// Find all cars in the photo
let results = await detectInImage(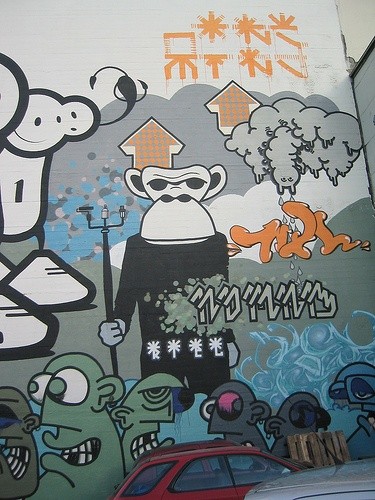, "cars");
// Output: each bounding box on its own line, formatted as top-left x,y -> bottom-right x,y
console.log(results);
242,458 -> 375,500
106,440 -> 309,500
